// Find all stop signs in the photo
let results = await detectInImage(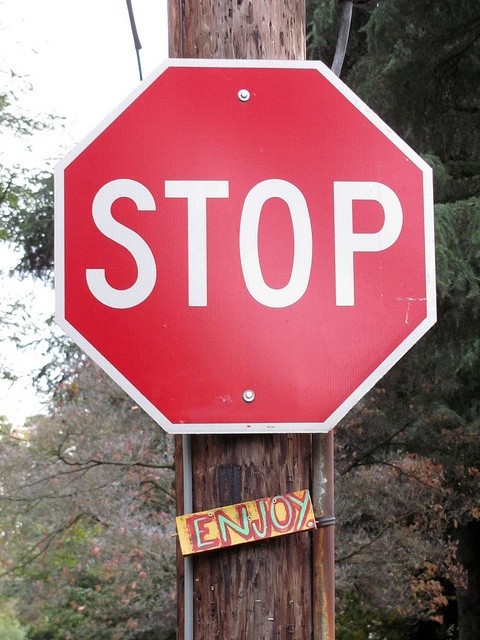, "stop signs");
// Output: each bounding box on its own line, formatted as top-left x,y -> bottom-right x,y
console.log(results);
54,59 -> 437,435
